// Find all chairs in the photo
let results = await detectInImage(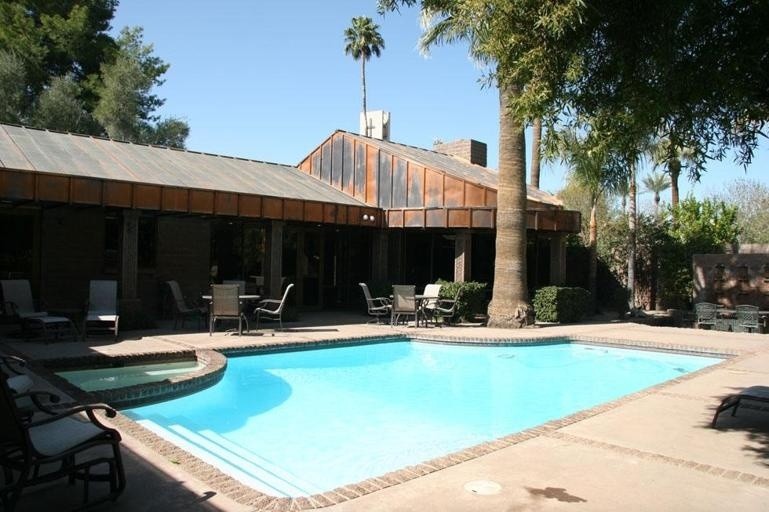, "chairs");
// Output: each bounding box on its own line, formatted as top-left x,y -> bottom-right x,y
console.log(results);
677,301 -> 769,334
0,278 -> 119,344
166,279 -> 295,335
712,386 -> 769,427
359,281 -> 463,328
0,353 -> 126,512
